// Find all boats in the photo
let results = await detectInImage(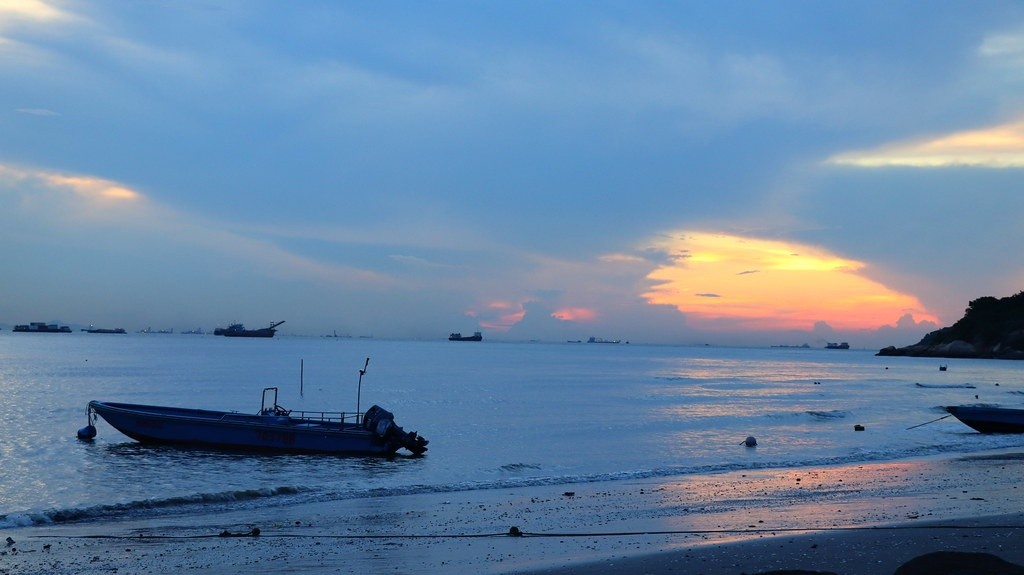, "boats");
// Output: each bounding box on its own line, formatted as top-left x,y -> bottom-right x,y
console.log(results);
213,320 -> 287,338
587,336 -> 621,343
90,386 -> 431,459
568,339 -> 581,343
12,322 -> 128,334
942,404 -> 1024,433
448,331 -> 483,342
